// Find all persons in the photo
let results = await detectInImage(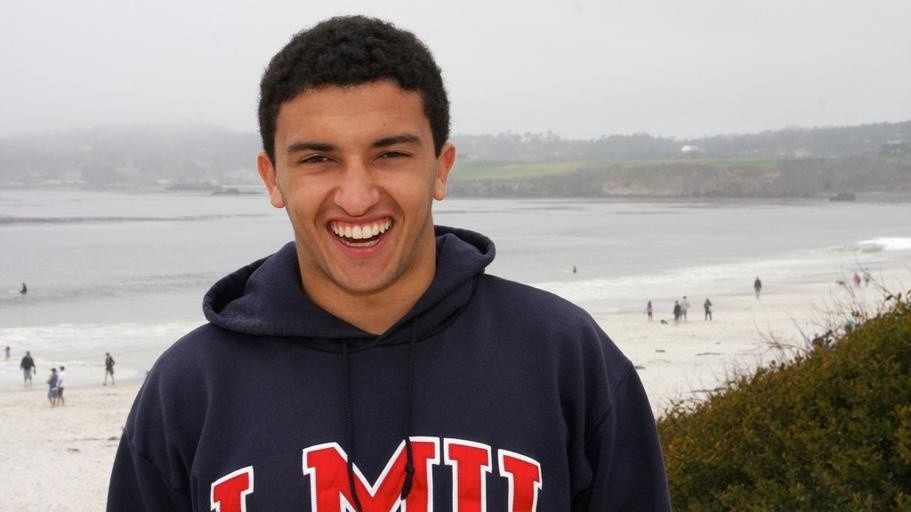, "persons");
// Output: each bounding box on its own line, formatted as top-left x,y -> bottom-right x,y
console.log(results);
704,299 -> 712,321
674,301 -> 681,320
643,302 -> 653,321
106,15 -> 672,512
103,353 -> 115,385
48,368 -> 56,407
680,297 -> 689,320
20,283 -> 27,294
754,277 -> 761,299
864,268 -> 871,287
56,367 -> 65,406
20,352 -> 35,389
853,272 -> 861,286
